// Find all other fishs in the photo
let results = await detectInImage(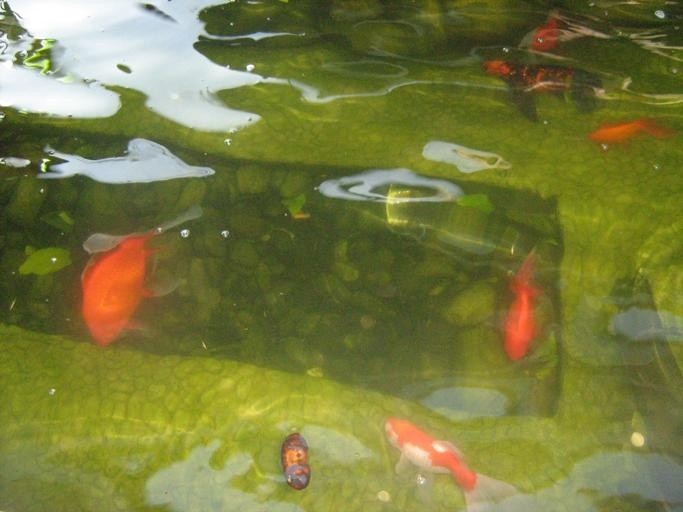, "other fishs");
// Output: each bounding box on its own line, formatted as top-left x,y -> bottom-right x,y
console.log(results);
79,200 -> 205,348
